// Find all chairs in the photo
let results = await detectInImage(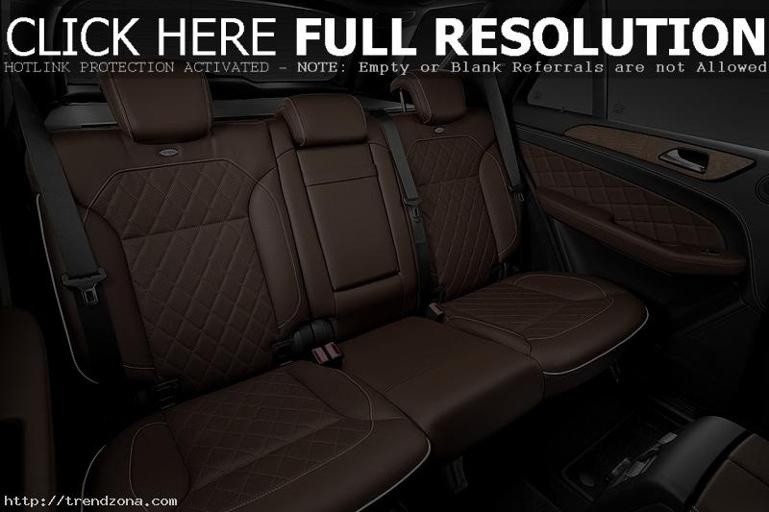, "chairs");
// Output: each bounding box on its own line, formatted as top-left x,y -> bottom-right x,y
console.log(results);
278,87 -> 545,478
34,60 -> 436,512
387,61 -> 651,398
